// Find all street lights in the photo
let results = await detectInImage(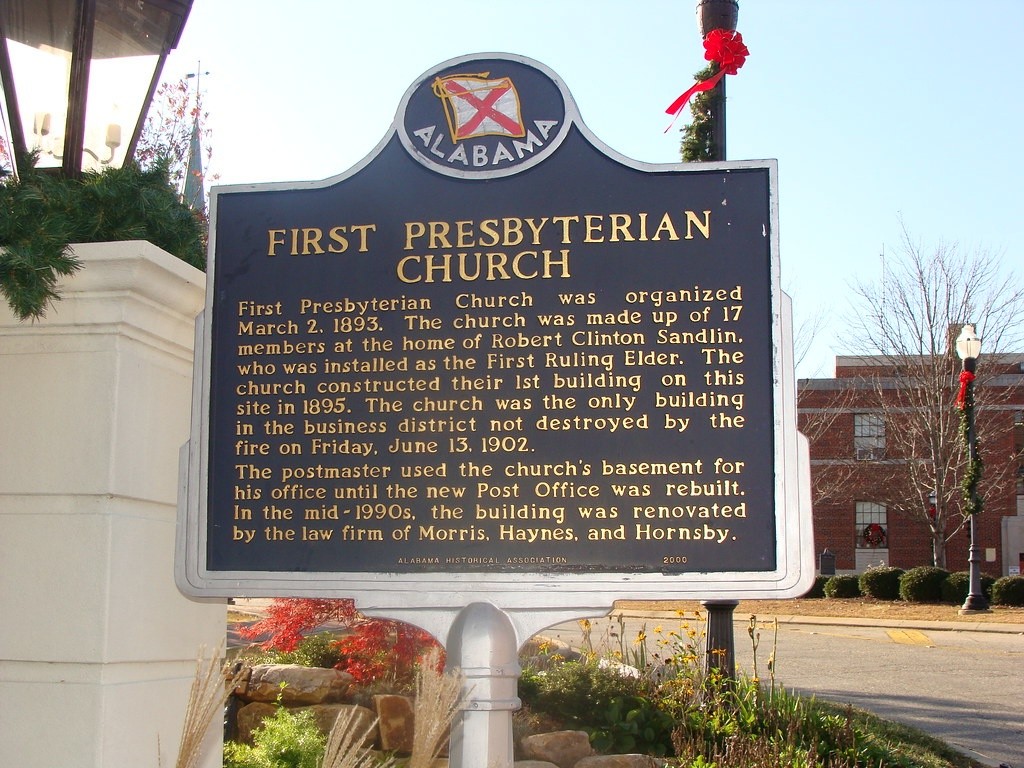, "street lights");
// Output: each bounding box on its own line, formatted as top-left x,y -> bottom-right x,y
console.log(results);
953,324 -> 992,614
